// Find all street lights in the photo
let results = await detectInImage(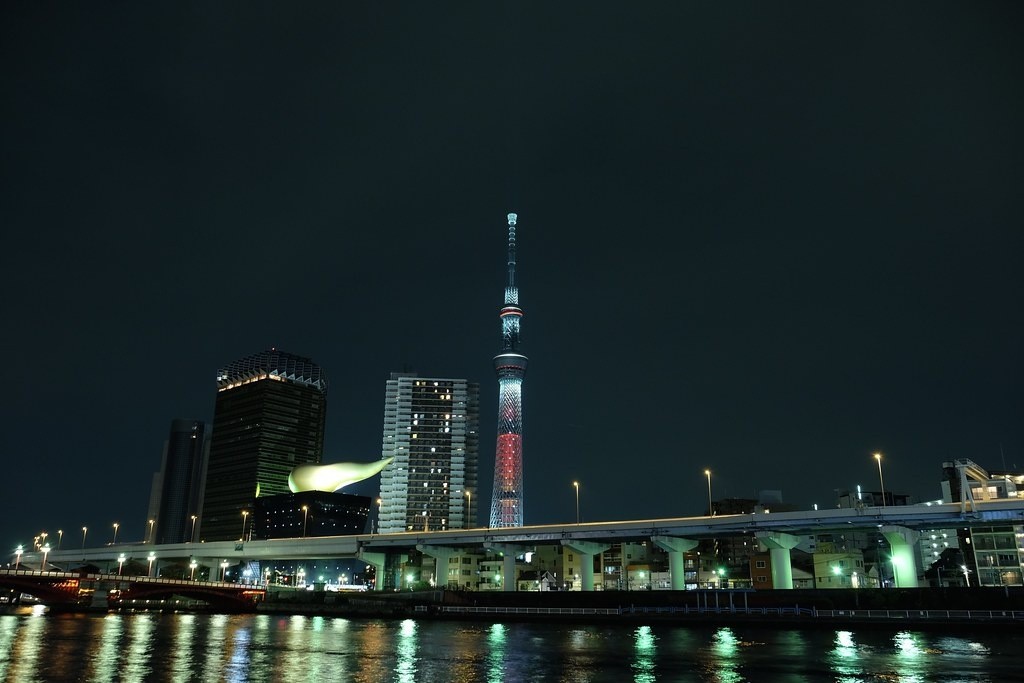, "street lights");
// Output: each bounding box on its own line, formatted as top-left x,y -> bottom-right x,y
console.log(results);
242,511 -> 248,540
190,560 -> 197,581
58,530 -> 63,550
221,563 -> 229,582
377,499 -> 381,534
117,554 -> 125,576
34,536 -> 41,552
113,523 -> 119,544
41,533 -> 48,545
147,552 -> 155,577
40,544 -> 50,572
303,506 -> 307,539
874,454 -> 886,506
465,491 -> 470,529
339,574 -> 348,588
192,515 -> 198,543
574,482 -> 579,523
16,544 -> 24,568
706,470 -> 711,516
83,527 -> 88,548
298,568 -> 306,586
